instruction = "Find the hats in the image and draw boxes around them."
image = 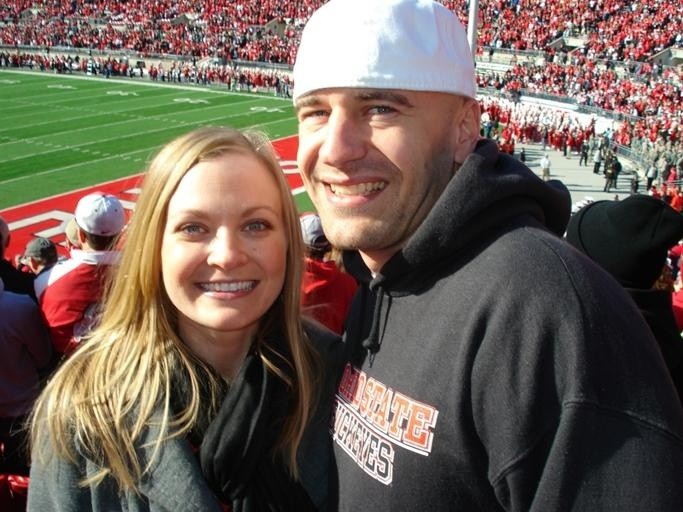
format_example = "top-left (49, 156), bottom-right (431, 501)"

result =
top-left (74, 193), bottom-right (123, 236)
top-left (64, 218), bottom-right (80, 247)
top-left (298, 214), bottom-right (332, 253)
top-left (566, 195), bottom-right (682, 289)
top-left (292, 0), bottom-right (476, 109)
top-left (24, 239), bottom-right (58, 264)
